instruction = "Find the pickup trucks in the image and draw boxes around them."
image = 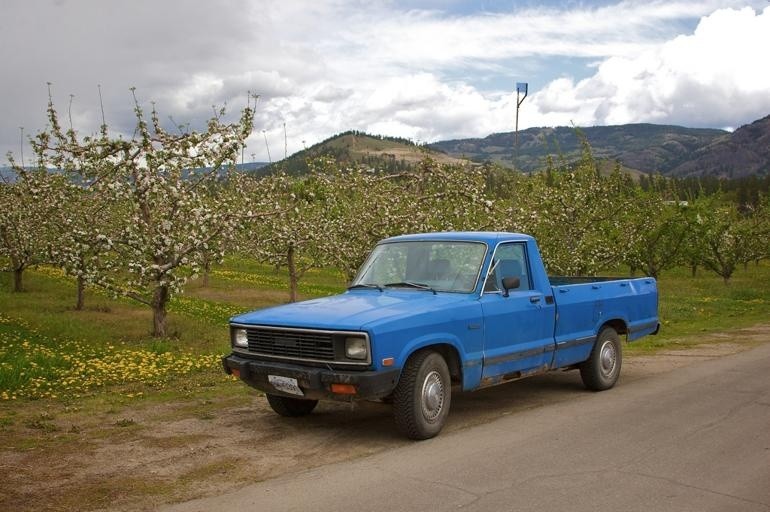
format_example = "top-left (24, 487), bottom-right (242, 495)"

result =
top-left (221, 230), bottom-right (660, 440)
